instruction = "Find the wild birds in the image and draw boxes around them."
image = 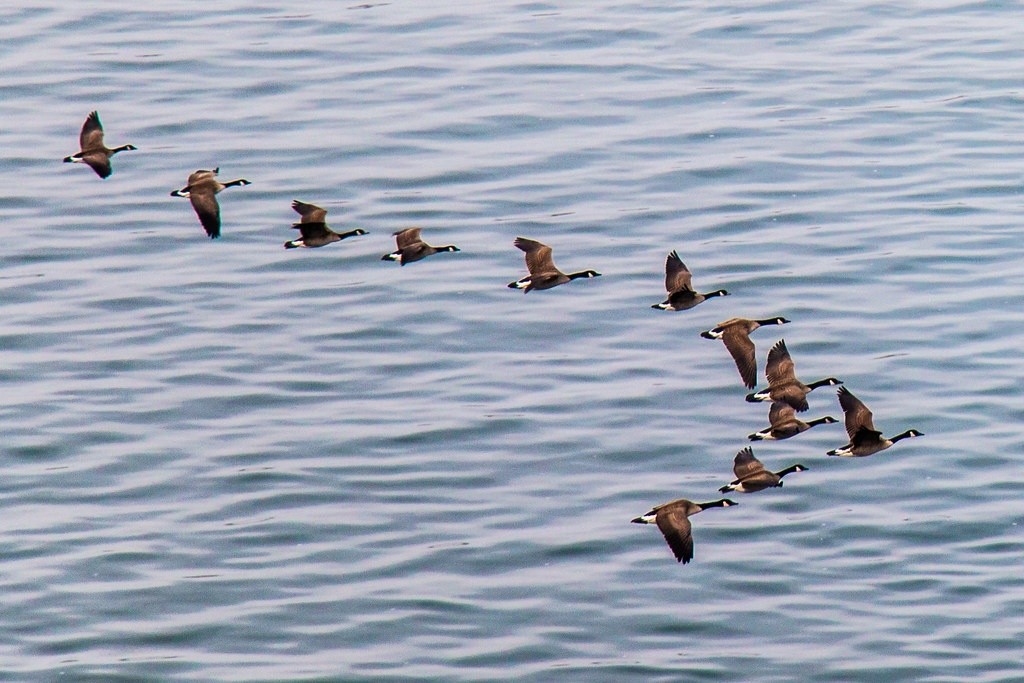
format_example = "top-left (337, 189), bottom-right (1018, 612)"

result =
top-left (700, 316), bottom-right (792, 390)
top-left (380, 227), bottom-right (460, 266)
top-left (747, 402), bottom-right (840, 443)
top-left (826, 386), bottom-right (925, 457)
top-left (63, 110), bottom-right (137, 180)
top-left (630, 499), bottom-right (739, 564)
top-left (650, 250), bottom-right (732, 311)
top-left (170, 167), bottom-right (252, 240)
top-left (718, 446), bottom-right (810, 495)
top-left (283, 198), bottom-right (370, 250)
top-left (507, 236), bottom-right (602, 295)
top-left (745, 338), bottom-right (844, 413)
